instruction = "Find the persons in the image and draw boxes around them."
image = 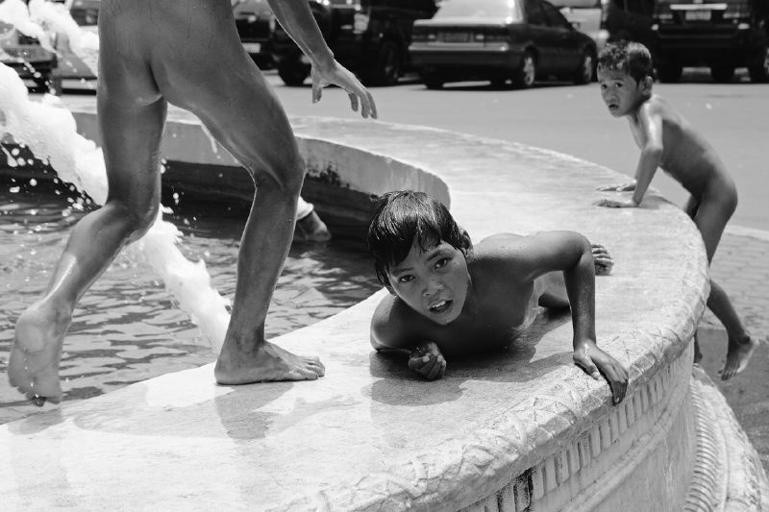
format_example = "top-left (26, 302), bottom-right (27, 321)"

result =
top-left (8, 0), bottom-right (377, 407)
top-left (595, 41), bottom-right (756, 380)
top-left (364, 191), bottom-right (628, 406)
